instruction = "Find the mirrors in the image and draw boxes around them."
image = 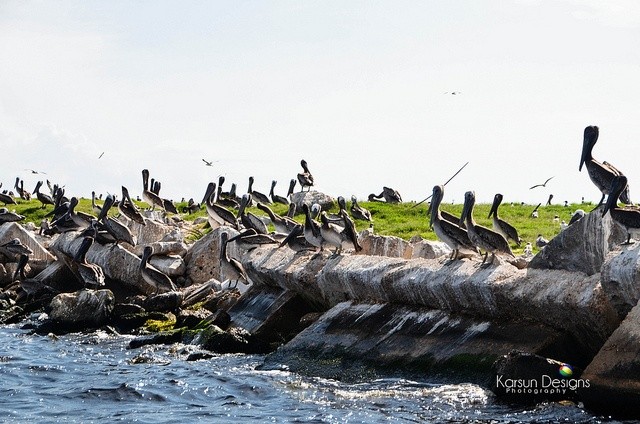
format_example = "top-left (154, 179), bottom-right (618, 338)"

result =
top-left (32, 181), bottom-right (56, 209)
top-left (55, 187), bottom-right (66, 210)
top-left (368, 189), bottom-right (404, 203)
top-left (427, 184), bottom-right (469, 231)
top-left (529, 176), bottom-right (555, 191)
top-left (524, 242), bottom-right (534, 258)
top-left (269, 180), bottom-right (290, 205)
top-left (546, 194), bottom-right (555, 206)
top-left (278, 224), bottom-right (321, 252)
top-left (359, 224), bottom-right (376, 242)
top-left (1, 238), bottom-right (34, 261)
top-left (219, 232), bottom-right (251, 291)
top-left (201, 182), bottom-right (238, 231)
top-left (46, 180), bottom-right (68, 196)
top-left (334, 196), bottom-right (364, 253)
top-left (429, 184), bottom-right (480, 268)
top-left (49, 197), bottom-right (99, 230)
top-left (553, 215), bottom-right (560, 225)
top-left (202, 159), bottom-right (215, 167)
top-left (14, 177), bottom-right (22, 195)
top-left (602, 175), bottom-right (640, 246)
top-left (216, 187), bottom-right (241, 210)
top-left (53, 184), bottom-right (70, 203)
top-left (320, 211), bottom-right (347, 260)
top-left (383, 186), bottom-right (403, 205)
top-left (257, 203), bottom-right (302, 235)
top-left (23, 169), bottom-right (48, 176)
top-left (248, 177), bottom-right (274, 206)
top-left (91, 192), bottom-right (103, 218)
top-left (219, 176), bottom-right (226, 187)
top-left (287, 202), bottom-right (298, 220)
top-left (9, 191), bottom-right (17, 203)
top-left (297, 160), bottom-right (316, 193)
top-left (458, 190), bottom-right (517, 270)
top-left (536, 234), bottom-right (548, 247)
top-left (569, 209), bottom-right (585, 226)
top-left (311, 203), bottom-right (322, 222)
top-left (227, 183), bottom-right (242, 206)
top-left (351, 195), bottom-right (375, 223)
top-left (226, 228), bottom-right (281, 248)
top-left (96, 194), bottom-right (136, 249)
top-left (19, 181), bottom-right (32, 202)
top-left (118, 186), bottom-right (148, 229)
top-left (564, 201), bottom-right (572, 207)
top-left (560, 221), bottom-right (568, 230)
top-left (151, 178), bottom-right (178, 215)
top-left (488, 194), bottom-right (523, 248)
top-left (287, 178), bottom-right (298, 198)
top-left (180, 197), bottom-right (201, 216)
top-left (71, 236), bottom-right (107, 293)
top-left (44, 202), bottom-right (81, 233)
top-left (237, 194), bottom-right (270, 235)
top-left (532, 203), bottom-right (542, 219)
top-left (13, 252), bottom-right (63, 304)
top-left (579, 125), bottom-right (635, 219)
top-left (0, 183), bottom-right (19, 208)
top-left (142, 169), bottom-right (166, 213)
top-left (138, 246), bottom-right (178, 297)
top-left (300, 203), bottom-right (329, 254)
